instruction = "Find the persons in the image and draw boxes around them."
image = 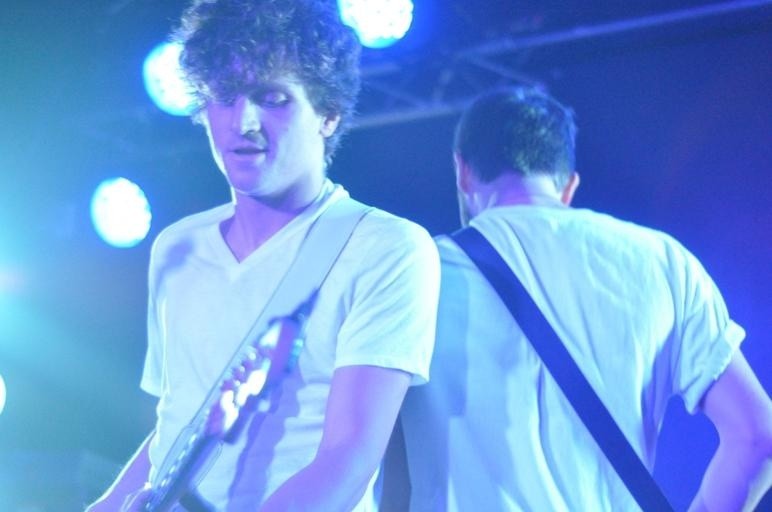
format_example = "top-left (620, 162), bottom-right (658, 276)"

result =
top-left (398, 87), bottom-right (770, 511)
top-left (79, 0), bottom-right (442, 512)
